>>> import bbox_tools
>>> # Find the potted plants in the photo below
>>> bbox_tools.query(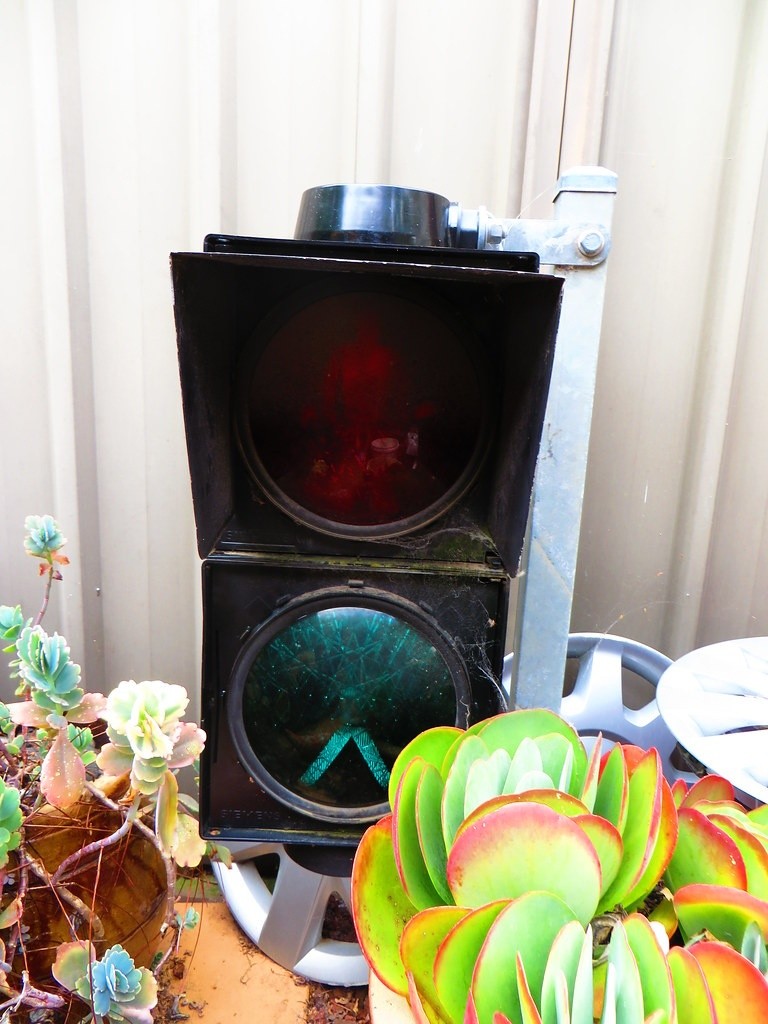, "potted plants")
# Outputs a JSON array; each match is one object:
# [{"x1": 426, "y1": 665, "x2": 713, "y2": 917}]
[
  {"x1": 352, "y1": 707, "x2": 768, "y2": 1024},
  {"x1": 0, "y1": 513, "x2": 232, "y2": 1024}
]
